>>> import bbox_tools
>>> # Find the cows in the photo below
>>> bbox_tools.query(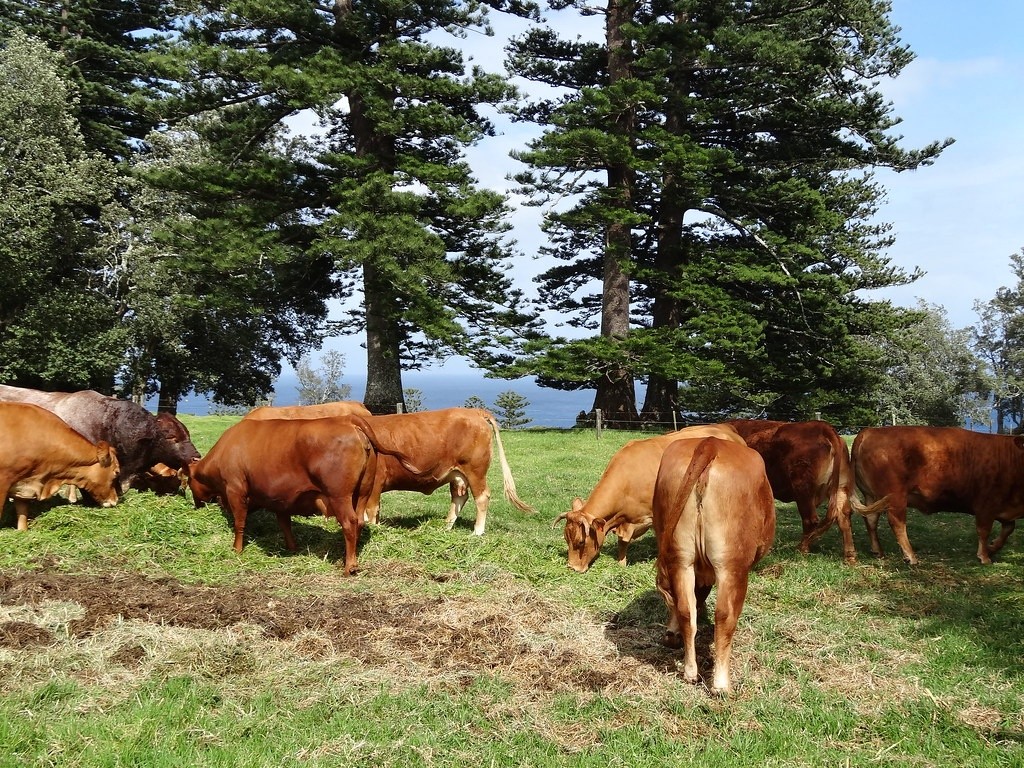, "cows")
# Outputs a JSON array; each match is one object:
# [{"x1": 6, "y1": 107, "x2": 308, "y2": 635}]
[
  {"x1": 551, "y1": 418, "x2": 1024, "y2": 699},
  {"x1": 177, "y1": 400, "x2": 535, "y2": 581},
  {"x1": 0, "y1": 385, "x2": 203, "y2": 532}
]
